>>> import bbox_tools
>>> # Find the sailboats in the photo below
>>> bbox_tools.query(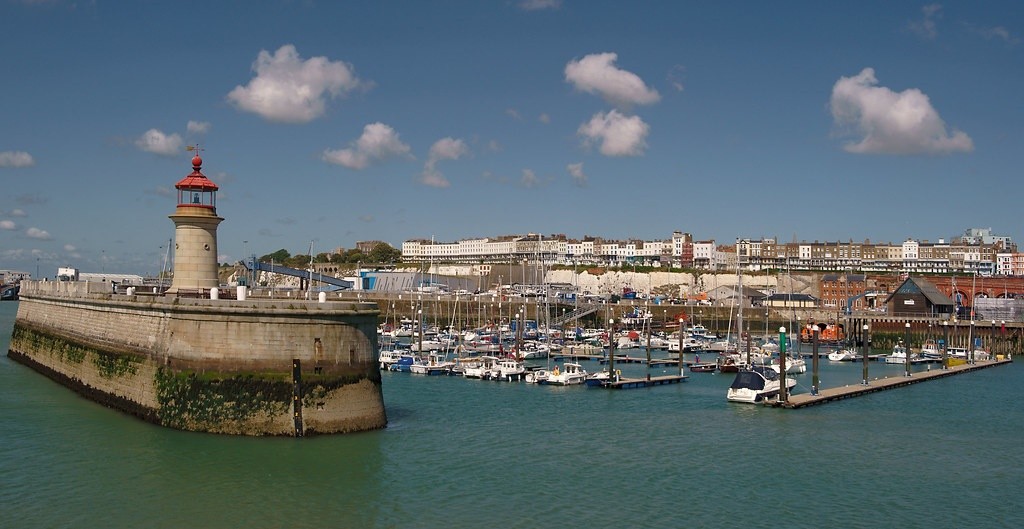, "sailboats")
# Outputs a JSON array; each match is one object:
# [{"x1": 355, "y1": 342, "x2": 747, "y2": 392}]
[{"x1": 226, "y1": 232, "x2": 992, "y2": 406}]
